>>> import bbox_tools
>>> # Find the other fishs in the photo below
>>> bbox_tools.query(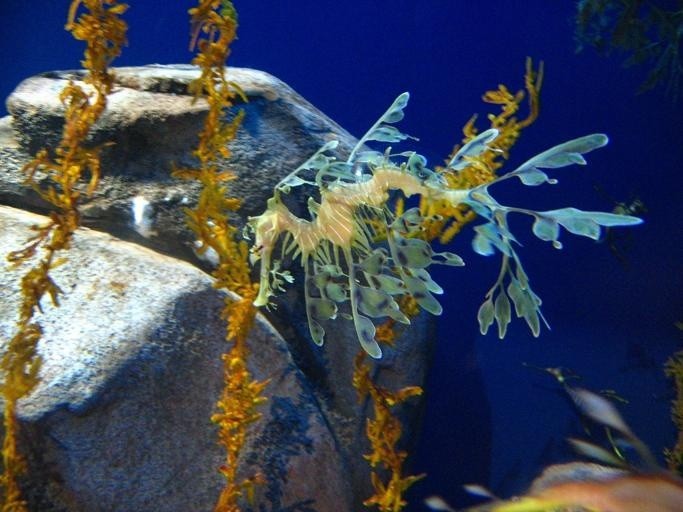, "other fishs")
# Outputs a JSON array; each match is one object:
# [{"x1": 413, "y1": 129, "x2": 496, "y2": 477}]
[
  {"x1": 559, "y1": 382, "x2": 657, "y2": 466},
  {"x1": 422, "y1": 432, "x2": 683, "y2": 511}
]
